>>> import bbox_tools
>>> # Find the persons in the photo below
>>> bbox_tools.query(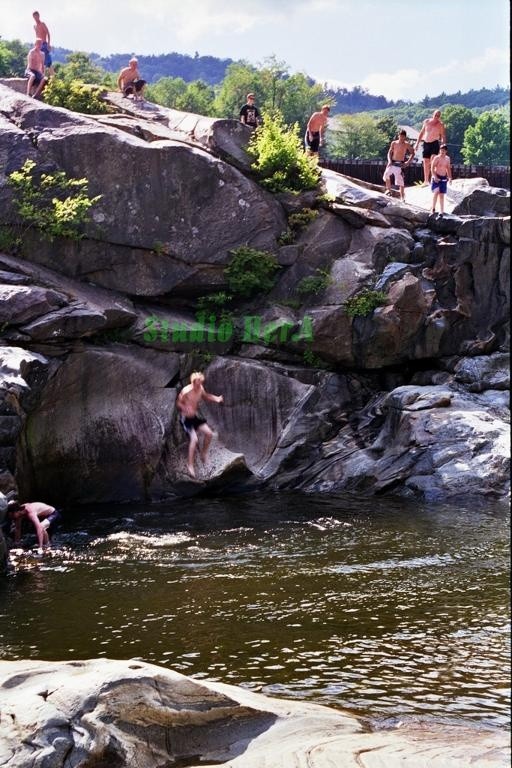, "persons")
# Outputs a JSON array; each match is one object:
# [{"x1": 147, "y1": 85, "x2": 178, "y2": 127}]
[
  {"x1": 239, "y1": 91, "x2": 259, "y2": 130},
  {"x1": 8, "y1": 497, "x2": 60, "y2": 554},
  {"x1": 414, "y1": 108, "x2": 448, "y2": 187},
  {"x1": 428, "y1": 143, "x2": 454, "y2": 221},
  {"x1": 382, "y1": 128, "x2": 416, "y2": 205},
  {"x1": 173, "y1": 368, "x2": 226, "y2": 478},
  {"x1": 117, "y1": 55, "x2": 147, "y2": 102},
  {"x1": 304, "y1": 102, "x2": 331, "y2": 161},
  {"x1": 31, "y1": 10, "x2": 57, "y2": 77},
  {"x1": 23, "y1": 37, "x2": 46, "y2": 98}
]
